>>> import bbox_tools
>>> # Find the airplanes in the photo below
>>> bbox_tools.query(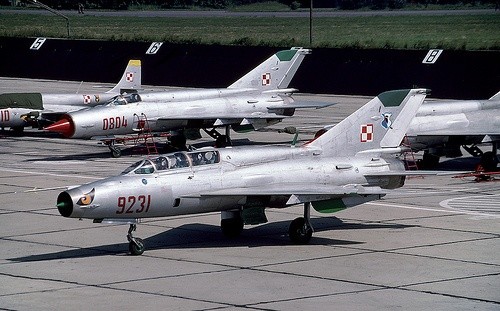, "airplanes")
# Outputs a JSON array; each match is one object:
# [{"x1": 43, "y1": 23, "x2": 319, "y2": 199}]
[
  {"x1": 55, "y1": 88, "x2": 434, "y2": 255},
  {"x1": 0, "y1": 59, "x2": 142, "y2": 137},
  {"x1": 43, "y1": 47, "x2": 341, "y2": 159},
  {"x1": 314, "y1": 89, "x2": 499, "y2": 171}
]
